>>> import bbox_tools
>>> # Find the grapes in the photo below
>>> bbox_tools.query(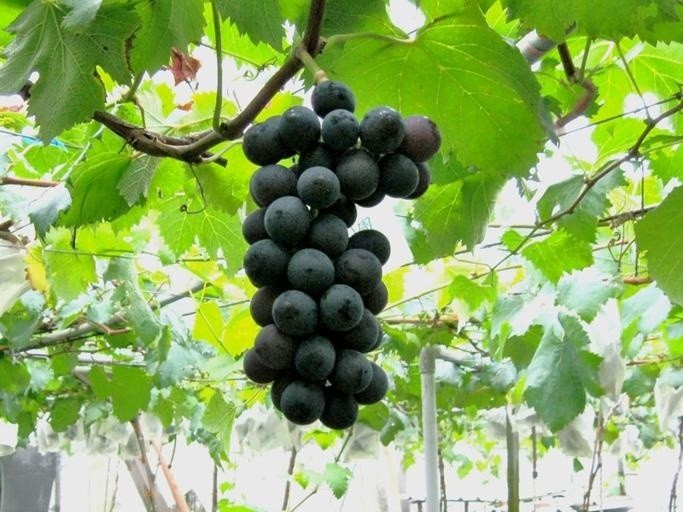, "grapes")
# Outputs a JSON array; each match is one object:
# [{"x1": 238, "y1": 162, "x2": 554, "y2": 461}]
[{"x1": 242, "y1": 80, "x2": 441, "y2": 431}]
